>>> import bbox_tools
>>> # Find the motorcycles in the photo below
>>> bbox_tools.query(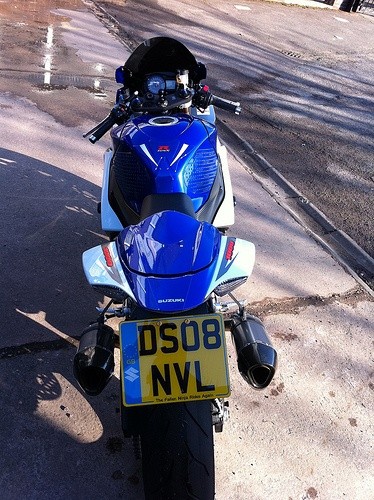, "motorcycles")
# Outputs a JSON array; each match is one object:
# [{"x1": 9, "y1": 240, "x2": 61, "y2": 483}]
[{"x1": 73, "y1": 37, "x2": 279, "y2": 500}]
